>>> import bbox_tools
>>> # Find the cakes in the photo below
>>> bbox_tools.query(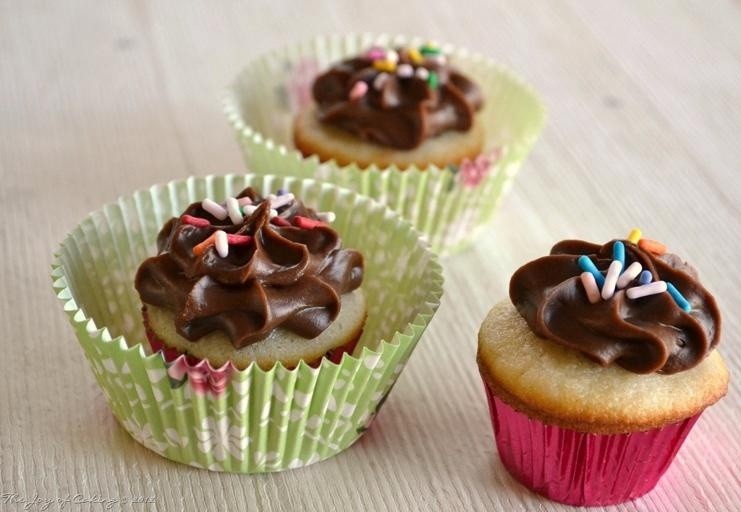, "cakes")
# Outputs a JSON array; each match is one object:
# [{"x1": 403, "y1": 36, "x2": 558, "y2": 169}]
[
  {"x1": 475, "y1": 227, "x2": 730, "y2": 433},
  {"x1": 135, "y1": 187, "x2": 367, "y2": 370},
  {"x1": 293, "y1": 44, "x2": 490, "y2": 170}
]
